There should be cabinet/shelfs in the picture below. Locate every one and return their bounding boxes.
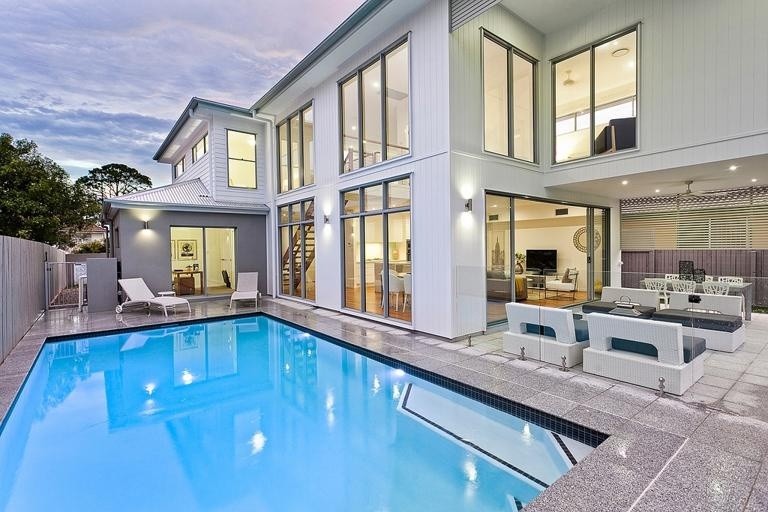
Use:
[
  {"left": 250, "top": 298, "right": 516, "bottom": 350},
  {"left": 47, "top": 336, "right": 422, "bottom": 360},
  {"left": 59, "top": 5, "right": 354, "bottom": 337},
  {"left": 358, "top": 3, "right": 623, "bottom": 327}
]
[{"left": 175, "top": 276, "right": 195, "bottom": 295}]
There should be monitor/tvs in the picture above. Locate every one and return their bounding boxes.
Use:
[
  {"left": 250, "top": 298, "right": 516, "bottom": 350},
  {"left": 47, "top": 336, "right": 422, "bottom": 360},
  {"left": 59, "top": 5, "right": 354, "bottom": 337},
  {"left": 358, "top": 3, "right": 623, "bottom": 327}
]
[{"left": 527, "top": 249, "right": 557, "bottom": 272}]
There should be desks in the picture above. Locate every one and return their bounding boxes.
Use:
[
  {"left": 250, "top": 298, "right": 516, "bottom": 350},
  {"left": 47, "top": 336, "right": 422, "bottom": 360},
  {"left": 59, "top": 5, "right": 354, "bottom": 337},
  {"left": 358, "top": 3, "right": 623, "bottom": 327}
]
[{"left": 174, "top": 271, "right": 204, "bottom": 294}]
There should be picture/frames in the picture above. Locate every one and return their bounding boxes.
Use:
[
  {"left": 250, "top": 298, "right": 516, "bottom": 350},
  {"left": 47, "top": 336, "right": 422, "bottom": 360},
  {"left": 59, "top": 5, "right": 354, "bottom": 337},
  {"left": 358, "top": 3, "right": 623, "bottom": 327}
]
[
  {"left": 171, "top": 240, "right": 177, "bottom": 261},
  {"left": 178, "top": 240, "right": 197, "bottom": 260}
]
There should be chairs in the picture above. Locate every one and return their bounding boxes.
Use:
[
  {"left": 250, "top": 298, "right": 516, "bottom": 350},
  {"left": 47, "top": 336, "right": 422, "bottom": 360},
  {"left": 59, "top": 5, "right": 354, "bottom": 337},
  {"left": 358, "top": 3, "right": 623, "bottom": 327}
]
[
  {"left": 379, "top": 268, "right": 412, "bottom": 313},
  {"left": 502, "top": 261, "right": 751, "bottom": 398},
  {"left": 116, "top": 279, "right": 192, "bottom": 320},
  {"left": 545, "top": 268, "right": 580, "bottom": 300},
  {"left": 593, "top": 116, "right": 635, "bottom": 156},
  {"left": 230, "top": 272, "right": 262, "bottom": 310}
]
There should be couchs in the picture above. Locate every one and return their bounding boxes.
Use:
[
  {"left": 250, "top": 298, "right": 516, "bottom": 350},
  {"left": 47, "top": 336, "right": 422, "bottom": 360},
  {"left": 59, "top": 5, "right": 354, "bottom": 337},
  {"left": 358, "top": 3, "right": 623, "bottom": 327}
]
[{"left": 486, "top": 271, "right": 528, "bottom": 302}]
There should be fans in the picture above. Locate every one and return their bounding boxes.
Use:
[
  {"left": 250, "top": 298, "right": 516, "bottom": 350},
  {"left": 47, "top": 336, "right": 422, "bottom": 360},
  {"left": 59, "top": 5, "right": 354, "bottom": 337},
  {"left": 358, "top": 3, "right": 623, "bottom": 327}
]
[
  {"left": 562, "top": 70, "right": 574, "bottom": 87},
  {"left": 662, "top": 179, "right": 729, "bottom": 200}
]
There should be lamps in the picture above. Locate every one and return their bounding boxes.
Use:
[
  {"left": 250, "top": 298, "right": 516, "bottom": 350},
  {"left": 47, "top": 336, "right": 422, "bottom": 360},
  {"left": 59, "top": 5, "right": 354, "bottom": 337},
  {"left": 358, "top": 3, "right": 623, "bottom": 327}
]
[
  {"left": 465, "top": 198, "right": 473, "bottom": 212},
  {"left": 144, "top": 222, "right": 148, "bottom": 229}
]
